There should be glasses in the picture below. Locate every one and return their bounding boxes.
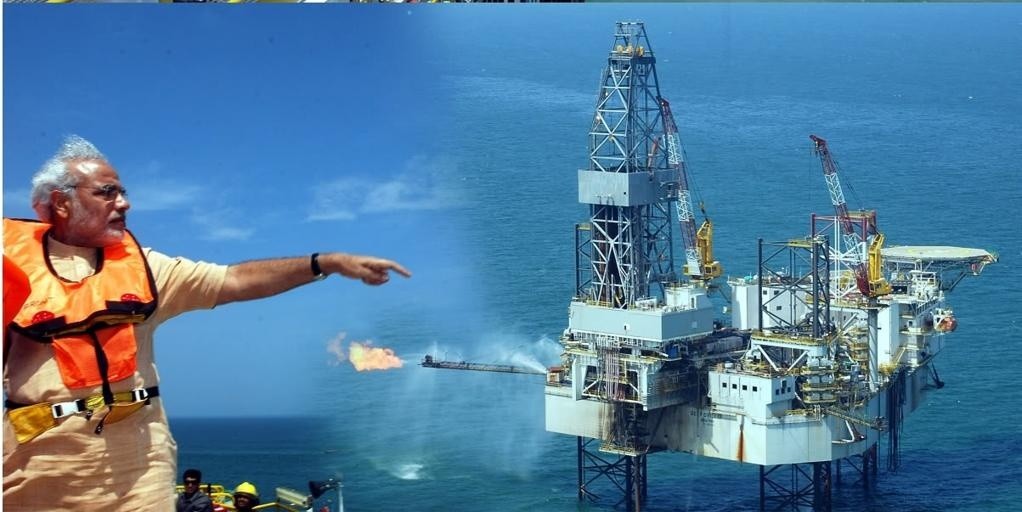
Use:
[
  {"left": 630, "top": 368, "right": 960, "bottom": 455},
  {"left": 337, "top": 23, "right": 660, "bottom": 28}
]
[{"left": 62, "top": 183, "right": 128, "bottom": 202}]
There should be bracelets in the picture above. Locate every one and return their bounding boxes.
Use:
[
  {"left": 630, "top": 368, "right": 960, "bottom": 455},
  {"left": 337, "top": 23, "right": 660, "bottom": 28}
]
[{"left": 310, "top": 252, "right": 328, "bottom": 280}]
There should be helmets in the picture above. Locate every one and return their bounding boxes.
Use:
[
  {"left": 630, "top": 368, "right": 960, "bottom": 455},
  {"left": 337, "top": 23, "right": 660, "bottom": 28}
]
[{"left": 234, "top": 482, "right": 257, "bottom": 497}]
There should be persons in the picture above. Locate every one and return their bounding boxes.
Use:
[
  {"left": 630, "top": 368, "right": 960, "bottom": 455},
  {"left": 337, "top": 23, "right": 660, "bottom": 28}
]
[
  {"left": 230, "top": 481, "right": 258, "bottom": 511},
  {"left": 4, "top": 134, "right": 411, "bottom": 512},
  {"left": 175, "top": 469, "right": 213, "bottom": 512}
]
